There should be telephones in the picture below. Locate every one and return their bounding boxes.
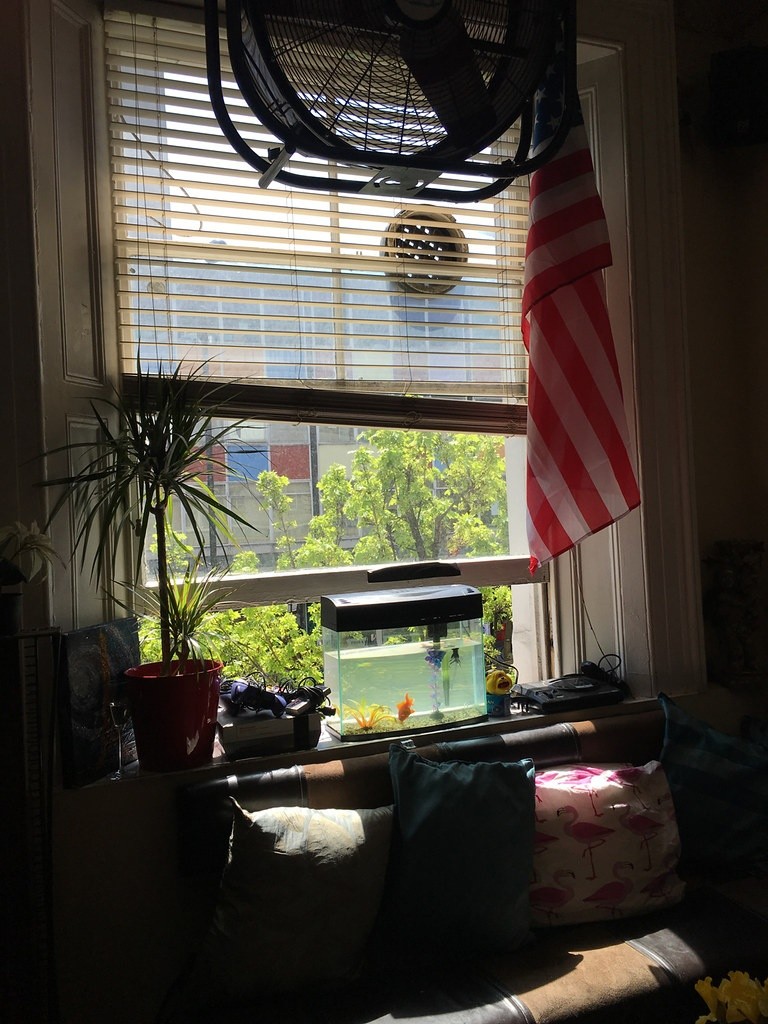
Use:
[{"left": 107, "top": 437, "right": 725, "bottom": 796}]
[{"left": 512, "top": 661, "right": 631, "bottom": 712}]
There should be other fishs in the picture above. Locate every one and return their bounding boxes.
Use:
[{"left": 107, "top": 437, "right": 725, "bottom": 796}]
[
  {"left": 397, "top": 692, "right": 416, "bottom": 721},
  {"left": 449, "top": 648, "right": 461, "bottom": 666}
]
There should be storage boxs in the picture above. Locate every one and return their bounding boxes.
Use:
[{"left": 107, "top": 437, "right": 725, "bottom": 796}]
[{"left": 320, "top": 583, "right": 488, "bottom": 742}]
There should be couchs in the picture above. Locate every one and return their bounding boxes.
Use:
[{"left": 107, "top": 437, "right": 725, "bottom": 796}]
[{"left": 172, "top": 708, "right": 767, "bottom": 1024}]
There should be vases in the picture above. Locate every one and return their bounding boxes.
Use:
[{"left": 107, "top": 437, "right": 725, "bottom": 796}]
[{"left": 0, "top": 593, "right": 25, "bottom": 634}]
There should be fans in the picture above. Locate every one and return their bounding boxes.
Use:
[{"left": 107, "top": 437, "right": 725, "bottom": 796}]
[{"left": 204, "top": 1, "right": 576, "bottom": 202}]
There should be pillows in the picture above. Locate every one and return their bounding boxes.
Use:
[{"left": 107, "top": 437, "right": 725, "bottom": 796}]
[
  {"left": 529, "top": 761, "right": 685, "bottom": 923},
  {"left": 389, "top": 741, "right": 538, "bottom": 948},
  {"left": 209, "top": 800, "right": 395, "bottom": 984}
]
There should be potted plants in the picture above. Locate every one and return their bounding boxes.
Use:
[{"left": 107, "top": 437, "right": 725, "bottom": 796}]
[
  {"left": 28, "top": 346, "right": 271, "bottom": 772},
  {"left": 483, "top": 585, "right": 513, "bottom": 640}
]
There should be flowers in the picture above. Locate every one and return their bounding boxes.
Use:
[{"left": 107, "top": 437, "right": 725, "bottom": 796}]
[
  {"left": 693, "top": 970, "right": 768, "bottom": 1024},
  {"left": 0, "top": 521, "right": 68, "bottom": 592}
]
